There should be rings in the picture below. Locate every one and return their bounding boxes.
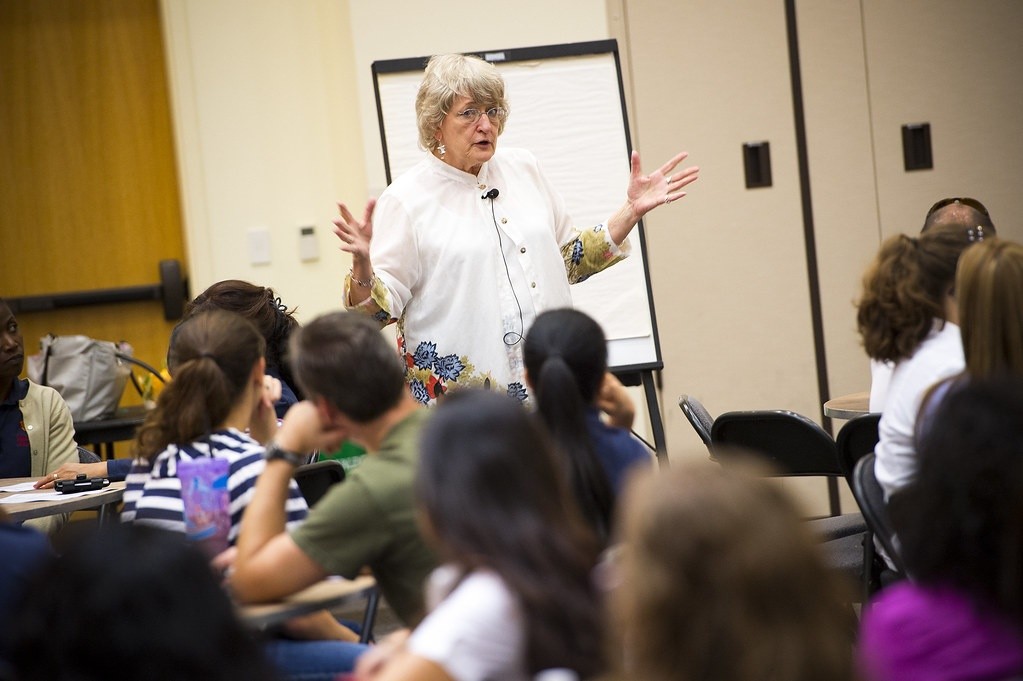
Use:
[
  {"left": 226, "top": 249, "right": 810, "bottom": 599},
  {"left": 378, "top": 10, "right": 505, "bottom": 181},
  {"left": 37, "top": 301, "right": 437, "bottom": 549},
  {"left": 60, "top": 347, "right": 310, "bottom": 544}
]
[
  {"left": 665, "top": 196, "right": 671, "bottom": 204},
  {"left": 54, "top": 474, "right": 57, "bottom": 479},
  {"left": 666, "top": 176, "right": 672, "bottom": 184}
]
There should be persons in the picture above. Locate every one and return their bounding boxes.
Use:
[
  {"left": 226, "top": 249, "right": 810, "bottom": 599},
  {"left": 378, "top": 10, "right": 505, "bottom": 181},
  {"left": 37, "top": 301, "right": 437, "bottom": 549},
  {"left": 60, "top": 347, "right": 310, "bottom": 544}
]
[
  {"left": 0, "top": 280, "right": 856, "bottom": 681},
  {"left": 333, "top": 54, "right": 699, "bottom": 411},
  {"left": 856, "top": 198, "right": 1023, "bottom": 681}
]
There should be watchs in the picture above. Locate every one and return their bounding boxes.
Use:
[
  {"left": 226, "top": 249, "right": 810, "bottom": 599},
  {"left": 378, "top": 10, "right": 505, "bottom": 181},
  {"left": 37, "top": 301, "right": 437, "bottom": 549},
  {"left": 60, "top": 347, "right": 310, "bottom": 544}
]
[{"left": 263, "top": 441, "right": 302, "bottom": 463}]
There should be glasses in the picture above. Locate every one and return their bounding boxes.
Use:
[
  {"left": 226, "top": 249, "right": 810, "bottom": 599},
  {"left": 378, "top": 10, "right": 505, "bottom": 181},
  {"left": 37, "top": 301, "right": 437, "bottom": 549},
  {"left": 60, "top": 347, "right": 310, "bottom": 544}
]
[{"left": 446, "top": 107, "right": 504, "bottom": 124}]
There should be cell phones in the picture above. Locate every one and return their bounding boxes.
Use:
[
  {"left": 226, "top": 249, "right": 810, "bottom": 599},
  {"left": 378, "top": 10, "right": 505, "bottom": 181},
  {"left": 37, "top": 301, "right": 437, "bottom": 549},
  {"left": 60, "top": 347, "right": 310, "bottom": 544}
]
[{"left": 55, "top": 479, "right": 110, "bottom": 493}]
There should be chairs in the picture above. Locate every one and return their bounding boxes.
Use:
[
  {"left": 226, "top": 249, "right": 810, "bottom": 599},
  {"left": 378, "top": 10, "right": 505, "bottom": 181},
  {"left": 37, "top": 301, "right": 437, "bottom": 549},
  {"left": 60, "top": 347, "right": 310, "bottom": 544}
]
[
  {"left": 294, "top": 460, "right": 390, "bottom": 651},
  {"left": 678, "top": 396, "right": 907, "bottom": 645}
]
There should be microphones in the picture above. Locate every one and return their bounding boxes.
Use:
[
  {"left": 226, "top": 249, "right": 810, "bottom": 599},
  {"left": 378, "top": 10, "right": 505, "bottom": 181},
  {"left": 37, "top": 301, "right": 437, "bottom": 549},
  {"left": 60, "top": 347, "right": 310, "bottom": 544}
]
[{"left": 487, "top": 189, "right": 499, "bottom": 199}]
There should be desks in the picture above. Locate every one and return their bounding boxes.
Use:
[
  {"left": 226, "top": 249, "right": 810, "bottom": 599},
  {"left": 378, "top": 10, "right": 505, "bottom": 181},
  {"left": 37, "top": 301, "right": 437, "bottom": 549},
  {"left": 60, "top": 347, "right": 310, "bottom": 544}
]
[
  {"left": 0, "top": 477, "right": 125, "bottom": 538},
  {"left": 822, "top": 394, "right": 870, "bottom": 420},
  {"left": 239, "top": 573, "right": 381, "bottom": 633},
  {"left": 69, "top": 404, "right": 159, "bottom": 463}
]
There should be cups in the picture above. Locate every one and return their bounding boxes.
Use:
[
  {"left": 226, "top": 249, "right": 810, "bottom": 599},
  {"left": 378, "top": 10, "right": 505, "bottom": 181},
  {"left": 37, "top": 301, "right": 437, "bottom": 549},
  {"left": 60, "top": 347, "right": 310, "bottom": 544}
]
[{"left": 176, "top": 458, "right": 231, "bottom": 557}]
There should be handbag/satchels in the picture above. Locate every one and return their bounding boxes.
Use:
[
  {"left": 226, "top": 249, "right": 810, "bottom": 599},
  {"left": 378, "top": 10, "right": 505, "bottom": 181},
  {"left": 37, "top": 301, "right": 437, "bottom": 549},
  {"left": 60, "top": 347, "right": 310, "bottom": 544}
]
[{"left": 25, "top": 332, "right": 168, "bottom": 423}]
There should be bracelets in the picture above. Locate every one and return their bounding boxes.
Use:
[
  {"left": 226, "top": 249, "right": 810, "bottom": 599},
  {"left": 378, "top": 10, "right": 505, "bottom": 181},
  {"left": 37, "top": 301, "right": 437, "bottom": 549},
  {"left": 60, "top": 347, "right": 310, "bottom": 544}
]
[{"left": 350, "top": 269, "right": 376, "bottom": 287}]
